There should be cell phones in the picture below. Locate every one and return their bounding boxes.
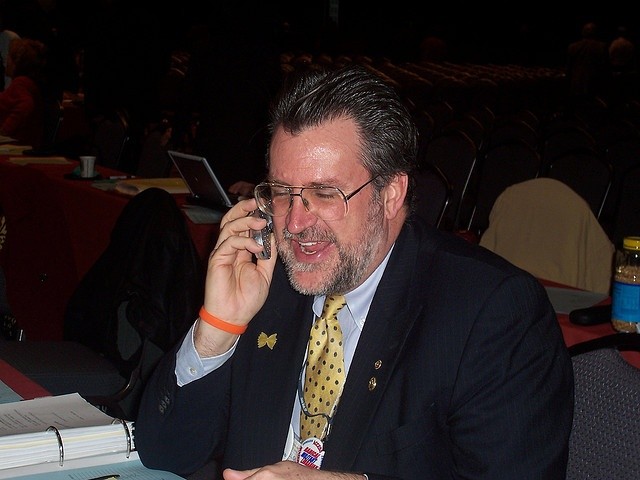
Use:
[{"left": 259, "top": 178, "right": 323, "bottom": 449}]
[{"left": 248, "top": 199, "right": 273, "bottom": 260}]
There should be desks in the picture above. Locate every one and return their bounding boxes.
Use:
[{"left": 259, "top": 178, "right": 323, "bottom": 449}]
[
  {"left": 539, "top": 276, "right": 640, "bottom": 372},
  {"left": 1, "top": 133, "right": 222, "bottom": 342},
  {"left": 0, "top": 362, "right": 53, "bottom": 400}
]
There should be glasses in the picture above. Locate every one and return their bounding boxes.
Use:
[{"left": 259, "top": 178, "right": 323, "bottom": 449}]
[{"left": 254, "top": 172, "right": 384, "bottom": 221}]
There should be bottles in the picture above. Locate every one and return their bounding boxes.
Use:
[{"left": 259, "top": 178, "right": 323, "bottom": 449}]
[{"left": 612, "top": 236, "right": 640, "bottom": 333}]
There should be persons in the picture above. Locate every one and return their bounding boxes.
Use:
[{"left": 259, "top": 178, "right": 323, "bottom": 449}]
[
  {"left": 134, "top": 65, "right": 574, "bottom": 478},
  {"left": 0, "top": 39, "right": 47, "bottom": 136},
  {"left": 23, "top": 68, "right": 126, "bottom": 170}
]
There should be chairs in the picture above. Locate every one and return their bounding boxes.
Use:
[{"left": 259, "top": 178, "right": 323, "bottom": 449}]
[
  {"left": 278, "top": 50, "right": 595, "bottom": 104},
  {"left": 2, "top": 337, "right": 147, "bottom": 397},
  {"left": 564, "top": 347, "right": 640, "bottom": 480},
  {"left": 405, "top": 99, "right": 629, "bottom": 231}
]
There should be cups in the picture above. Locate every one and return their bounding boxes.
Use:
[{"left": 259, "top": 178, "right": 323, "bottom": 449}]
[{"left": 79, "top": 155, "right": 96, "bottom": 180}]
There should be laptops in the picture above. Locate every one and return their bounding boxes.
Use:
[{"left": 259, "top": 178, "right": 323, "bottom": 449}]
[{"left": 168, "top": 150, "right": 254, "bottom": 211}]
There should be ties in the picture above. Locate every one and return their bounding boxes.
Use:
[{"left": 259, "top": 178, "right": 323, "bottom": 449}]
[{"left": 300, "top": 296, "right": 346, "bottom": 443}]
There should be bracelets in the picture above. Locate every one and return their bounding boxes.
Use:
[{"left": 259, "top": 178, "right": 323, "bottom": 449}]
[{"left": 199, "top": 307, "right": 251, "bottom": 336}]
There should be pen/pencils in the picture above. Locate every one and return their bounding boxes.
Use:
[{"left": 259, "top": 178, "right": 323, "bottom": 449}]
[
  {"left": 107, "top": 175, "right": 136, "bottom": 180},
  {"left": 86, "top": 474, "right": 120, "bottom": 480}
]
